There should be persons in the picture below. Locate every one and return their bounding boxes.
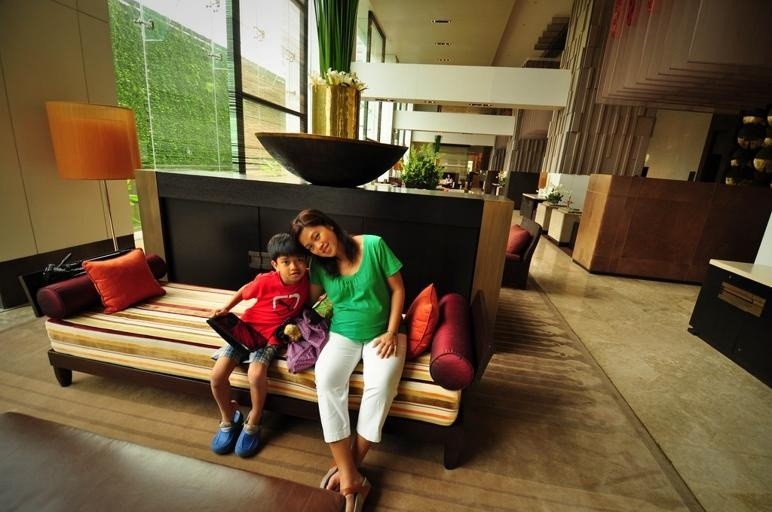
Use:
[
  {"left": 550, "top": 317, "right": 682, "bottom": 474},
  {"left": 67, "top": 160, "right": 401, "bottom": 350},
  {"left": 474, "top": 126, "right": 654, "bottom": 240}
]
[
  {"left": 207, "top": 235, "right": 315, "bottom": 458},
  {"left": 288, "top": 209, "right": 408, "bottom": 510}
]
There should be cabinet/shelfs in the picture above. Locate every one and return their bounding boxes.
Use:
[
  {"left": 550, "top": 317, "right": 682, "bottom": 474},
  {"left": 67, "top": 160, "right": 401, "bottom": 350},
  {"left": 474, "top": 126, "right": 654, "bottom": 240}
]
[
  {"left": 695, "top": 113, "right": 772, "bottom": 184},
  {"left": 687, "top": 258, "right": 772, "bottom": 388}
]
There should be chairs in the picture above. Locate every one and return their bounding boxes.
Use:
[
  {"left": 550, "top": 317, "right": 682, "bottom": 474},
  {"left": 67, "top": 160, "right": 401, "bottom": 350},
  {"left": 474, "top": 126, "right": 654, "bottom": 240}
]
[{"left": 501, "top": 217, "right": 542, "bottom": 289}]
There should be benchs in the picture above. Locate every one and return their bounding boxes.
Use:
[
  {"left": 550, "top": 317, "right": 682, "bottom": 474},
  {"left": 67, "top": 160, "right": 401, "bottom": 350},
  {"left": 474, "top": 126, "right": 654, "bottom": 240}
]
[{"left": 19, "top": 250, "right": 495, "bottom": 469}]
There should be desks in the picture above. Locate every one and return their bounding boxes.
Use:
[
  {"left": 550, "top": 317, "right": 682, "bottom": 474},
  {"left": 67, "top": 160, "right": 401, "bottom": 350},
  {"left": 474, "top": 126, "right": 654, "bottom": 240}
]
[{"left": 523, "top": 193, "right": 582, "bottom": 247}]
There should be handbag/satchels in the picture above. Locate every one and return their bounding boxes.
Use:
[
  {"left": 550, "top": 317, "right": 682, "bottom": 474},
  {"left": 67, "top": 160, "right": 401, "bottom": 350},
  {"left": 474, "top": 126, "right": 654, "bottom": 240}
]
[{"left": 287, "top": 309, "right": 329, "bottom": 373}]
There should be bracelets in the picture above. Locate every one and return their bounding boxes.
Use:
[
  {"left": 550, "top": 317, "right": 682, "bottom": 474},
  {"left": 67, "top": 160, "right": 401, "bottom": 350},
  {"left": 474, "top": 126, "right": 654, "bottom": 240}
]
[{"left": 386, "top": 331, "right": 397, "bottom": 336}]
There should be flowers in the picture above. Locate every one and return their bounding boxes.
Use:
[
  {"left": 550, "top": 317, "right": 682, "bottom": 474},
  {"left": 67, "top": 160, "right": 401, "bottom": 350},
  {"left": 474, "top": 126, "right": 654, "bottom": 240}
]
[
  {"left": 545, "top": 185, "right": 570, "bottom": 205},
  {"left": 311, "top": 67, "right": 368, "bottom": 91}
]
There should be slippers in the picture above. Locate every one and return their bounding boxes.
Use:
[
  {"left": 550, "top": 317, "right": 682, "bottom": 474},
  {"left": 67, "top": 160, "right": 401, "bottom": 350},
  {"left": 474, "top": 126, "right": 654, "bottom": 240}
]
[
  {"left": 320, "top": 465, "right": 338, "bottom": 490},
  {"left": 341, "top": 477, "right": 372, "bottom": 512},
  {"left": 235, "top": 410, "right": 265, "bottom": 457},
  {"left": 210, "top": 410, "right": 243, "bottom": 455}
]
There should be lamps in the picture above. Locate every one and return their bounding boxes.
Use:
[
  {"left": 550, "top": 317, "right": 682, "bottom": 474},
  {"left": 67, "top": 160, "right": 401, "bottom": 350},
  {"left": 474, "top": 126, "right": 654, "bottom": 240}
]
[{"left": 47, "top": 100, "right": 143, "bottom": 252}]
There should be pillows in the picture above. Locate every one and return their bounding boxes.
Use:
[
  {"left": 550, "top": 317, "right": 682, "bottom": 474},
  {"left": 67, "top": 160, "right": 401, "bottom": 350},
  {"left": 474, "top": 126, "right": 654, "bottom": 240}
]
[
  {"left": 507, "top": 225, "right": 530, "bottom": 254},
  {"left": 82, "top": 248, "right": 166, "bottom": 315},
  {"left": 37, "top": 254, "right": 166, "bottom": 320},
  {"left": 429, "top": 293, "right": 475, "bottom": 391},
  {"left": 404, "top": 283, "right": 441, "bottom": 361}
]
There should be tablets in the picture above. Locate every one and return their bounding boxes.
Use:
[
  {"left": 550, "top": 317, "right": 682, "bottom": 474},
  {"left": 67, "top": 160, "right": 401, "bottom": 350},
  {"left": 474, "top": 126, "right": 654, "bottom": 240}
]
[{"left": 206, "top": 312, "right": 269, "bottom": 352}]
